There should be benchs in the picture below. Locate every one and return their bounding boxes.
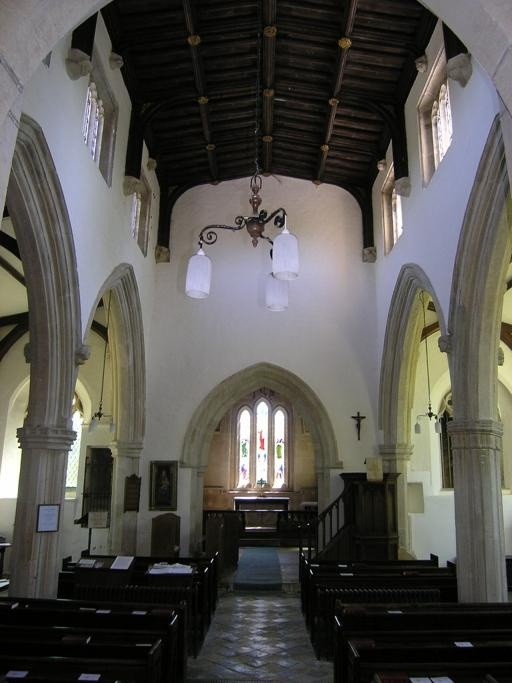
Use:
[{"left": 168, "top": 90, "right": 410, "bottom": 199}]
[
  {"left": 1, "top": 550, "right": 218, "bottom": 683},
  {"left": 298, "top": 552, "right": 512, "bottom": 682}
]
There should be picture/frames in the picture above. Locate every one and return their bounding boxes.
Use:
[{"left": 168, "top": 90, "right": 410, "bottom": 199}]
[{"left": 148, "top": 460, "right": 179, "bottom": 511}]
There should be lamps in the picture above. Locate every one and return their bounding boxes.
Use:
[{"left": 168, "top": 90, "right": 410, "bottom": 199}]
[
  {"left": 182, "top": 122, "right": 300, "bottom": 313},
  {"left": 87, "top": 290, "right": 118, "bottom": 434},
  {"left": 414, "top": 291, "right": 442, "bottom": 433}
]
